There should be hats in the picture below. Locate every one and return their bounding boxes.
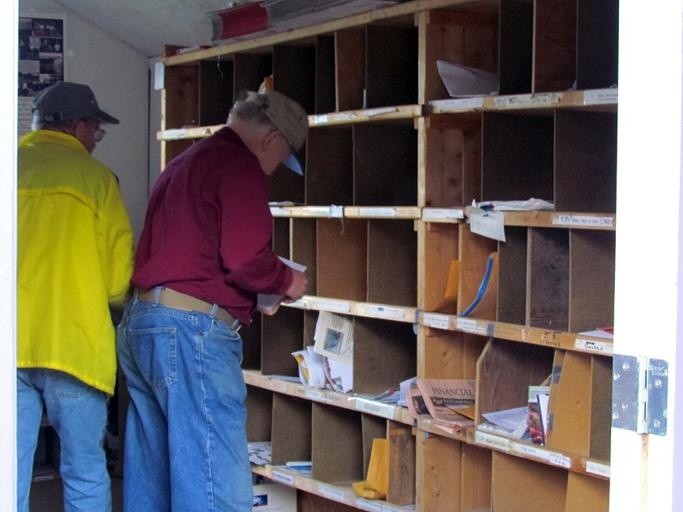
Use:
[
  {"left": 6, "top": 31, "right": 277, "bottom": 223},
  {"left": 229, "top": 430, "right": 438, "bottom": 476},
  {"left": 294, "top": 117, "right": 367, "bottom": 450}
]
[
  {"left": 249, "top": 91, "right": 309, "bottom": 176},
  {"left": 30, "top": 81, "right": 119, "bottom": 124}
]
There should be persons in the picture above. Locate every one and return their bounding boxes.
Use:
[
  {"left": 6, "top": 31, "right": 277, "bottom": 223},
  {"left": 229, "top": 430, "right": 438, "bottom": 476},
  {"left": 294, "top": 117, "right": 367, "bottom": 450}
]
[
  {"left": 39, "top": 37, "right": 51, "bottom": 54},
  {"left": 17, "top": 80, "right": 136, "bottom": 510},
  {"left": 111, "top": 88, "right": 312, "bottom": 511},
  {"left": 27, "top": 29, "right": 40, "bottom": 61}
]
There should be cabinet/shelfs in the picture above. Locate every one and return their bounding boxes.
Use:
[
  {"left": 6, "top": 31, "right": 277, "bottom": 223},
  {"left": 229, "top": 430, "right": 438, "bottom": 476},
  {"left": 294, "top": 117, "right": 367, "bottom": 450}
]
[{"left": 155, "top": 0, "right": 620, "bottom": 512}]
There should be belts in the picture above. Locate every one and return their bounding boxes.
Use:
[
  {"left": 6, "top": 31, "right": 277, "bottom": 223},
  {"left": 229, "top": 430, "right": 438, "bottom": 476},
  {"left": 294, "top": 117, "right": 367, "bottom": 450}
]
[{"left": 138, "top": 286, "right": 243, "bottom": 333}]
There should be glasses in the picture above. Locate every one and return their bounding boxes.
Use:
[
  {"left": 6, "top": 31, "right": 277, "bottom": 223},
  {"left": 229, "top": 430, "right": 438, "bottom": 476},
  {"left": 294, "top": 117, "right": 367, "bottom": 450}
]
[{"left": 94, "top": 126, "right": 105, "bottom": 142}]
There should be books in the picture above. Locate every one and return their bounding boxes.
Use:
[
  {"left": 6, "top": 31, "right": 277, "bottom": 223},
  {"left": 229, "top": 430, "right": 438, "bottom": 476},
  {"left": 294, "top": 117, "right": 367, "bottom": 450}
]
[{"left": 253, "top": 254, "right": 311, "bottom": 317}]
